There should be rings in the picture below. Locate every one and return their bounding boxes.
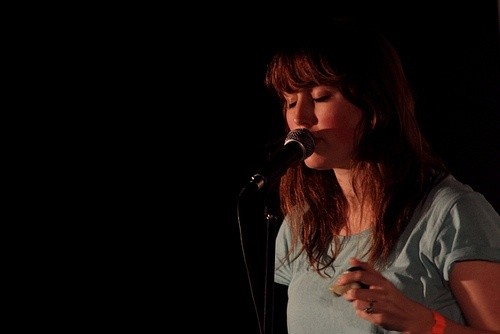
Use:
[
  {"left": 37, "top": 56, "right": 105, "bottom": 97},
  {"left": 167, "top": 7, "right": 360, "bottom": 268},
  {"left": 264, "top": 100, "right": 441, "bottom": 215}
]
[{"left": 364, "top": 303, "right": 377, "bottom": 313}]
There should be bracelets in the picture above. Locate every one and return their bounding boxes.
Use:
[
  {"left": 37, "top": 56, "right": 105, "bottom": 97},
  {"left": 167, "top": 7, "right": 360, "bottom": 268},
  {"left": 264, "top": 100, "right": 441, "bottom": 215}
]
[{"left": 432, "top": 309, "right": 446, "bottom": 334}]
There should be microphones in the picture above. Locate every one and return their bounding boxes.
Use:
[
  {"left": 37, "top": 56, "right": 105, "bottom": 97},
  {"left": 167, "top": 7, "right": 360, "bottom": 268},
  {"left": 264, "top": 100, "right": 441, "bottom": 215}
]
[{"left": 249, "top": 128, "right": 316, "bottom": 190}]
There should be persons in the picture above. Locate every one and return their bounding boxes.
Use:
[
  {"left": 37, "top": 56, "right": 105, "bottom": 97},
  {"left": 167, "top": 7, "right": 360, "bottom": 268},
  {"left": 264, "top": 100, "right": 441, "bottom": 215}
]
[{"left": 264, "top": 20, "right": 500, "bottom": 334}]
